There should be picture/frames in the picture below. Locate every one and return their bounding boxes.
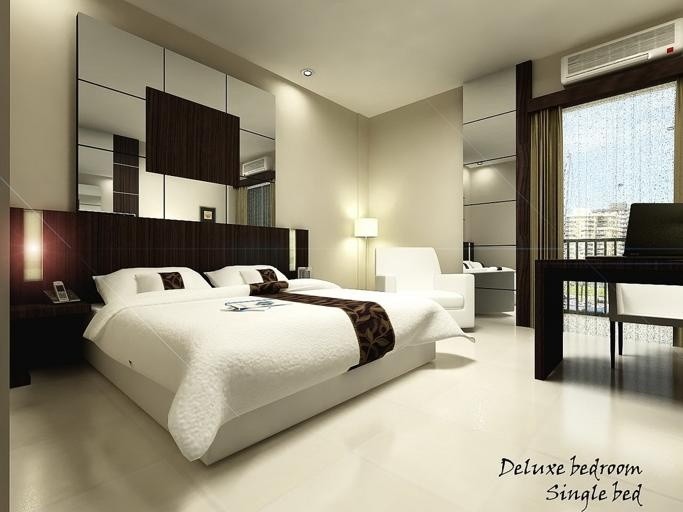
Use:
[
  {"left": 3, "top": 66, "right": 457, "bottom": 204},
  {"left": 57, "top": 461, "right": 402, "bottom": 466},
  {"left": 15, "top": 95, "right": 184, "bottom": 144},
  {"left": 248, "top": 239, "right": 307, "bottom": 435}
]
[{"left": 199, "top": 205, "right": 215, "bottom": 223}]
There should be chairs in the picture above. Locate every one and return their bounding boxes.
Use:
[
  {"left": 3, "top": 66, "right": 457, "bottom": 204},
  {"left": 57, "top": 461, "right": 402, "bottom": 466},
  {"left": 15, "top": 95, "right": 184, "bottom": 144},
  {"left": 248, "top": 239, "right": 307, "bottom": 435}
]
[
  {"left": 463, "top": 256, "right": 516, "bottom": 316},
  {"left": 372, "top": 243, "right": 475, "bottom": 330},
  {"left": 608, "top": 202, "right": 683, "bottom": 370}
]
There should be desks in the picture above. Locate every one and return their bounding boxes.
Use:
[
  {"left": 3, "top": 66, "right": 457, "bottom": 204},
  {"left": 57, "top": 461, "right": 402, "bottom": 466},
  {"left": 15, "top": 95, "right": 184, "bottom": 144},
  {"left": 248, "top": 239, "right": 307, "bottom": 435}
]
[
  {"left": 534, "top": 258, "right": 682, "bottom": 381},
  {"left": 10, "top": 300, "right": 91, "bottom": 390}
]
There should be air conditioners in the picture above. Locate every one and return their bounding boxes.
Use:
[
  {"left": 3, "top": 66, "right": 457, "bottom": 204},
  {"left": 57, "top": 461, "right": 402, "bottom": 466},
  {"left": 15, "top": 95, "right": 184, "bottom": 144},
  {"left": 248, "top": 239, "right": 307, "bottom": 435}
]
[
  {"left": 241, "top": 156, "right": 272, "bottom": 176},
  {"left": 559, "top": 16, "right": 683, "bottom": 89}
]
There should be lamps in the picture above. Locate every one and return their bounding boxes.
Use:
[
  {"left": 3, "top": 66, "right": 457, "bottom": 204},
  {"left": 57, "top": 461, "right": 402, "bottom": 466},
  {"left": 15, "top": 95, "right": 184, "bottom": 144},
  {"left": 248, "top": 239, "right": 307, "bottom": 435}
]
[{"left": 353, "top": 217, "right": 379, "bottom": 290}]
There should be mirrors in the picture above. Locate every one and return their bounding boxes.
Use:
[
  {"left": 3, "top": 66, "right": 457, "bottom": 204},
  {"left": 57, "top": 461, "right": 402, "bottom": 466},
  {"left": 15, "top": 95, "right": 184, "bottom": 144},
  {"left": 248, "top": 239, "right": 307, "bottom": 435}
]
[
  {"left": 73, "top": 10, "right": 278, "bottom": 228},
  {"left": 463, "top": 63, "right": 518, "bottom": 326}
]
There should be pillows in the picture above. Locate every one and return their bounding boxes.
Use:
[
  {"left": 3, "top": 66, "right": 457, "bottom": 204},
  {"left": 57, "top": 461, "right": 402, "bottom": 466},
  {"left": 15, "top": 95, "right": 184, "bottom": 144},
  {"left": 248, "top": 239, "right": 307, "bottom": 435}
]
[
  {"left": 201, "top": 263, "right": 289, "bottom": 288},
  {"left": 237, "top": 268, "right": 279, "bottom": 285},
  {"left": 132, "top": 271, "right": 185, "bottom": 295},
  {"left": 90, "top": 266, "right": 213, "bottom": 305}
]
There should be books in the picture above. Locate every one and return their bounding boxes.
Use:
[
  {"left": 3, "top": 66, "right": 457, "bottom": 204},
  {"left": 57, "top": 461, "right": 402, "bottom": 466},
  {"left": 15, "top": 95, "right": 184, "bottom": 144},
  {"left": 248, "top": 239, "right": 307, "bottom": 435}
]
[{"left": 221, "top": 298, "right": 288, "bottom": 310}]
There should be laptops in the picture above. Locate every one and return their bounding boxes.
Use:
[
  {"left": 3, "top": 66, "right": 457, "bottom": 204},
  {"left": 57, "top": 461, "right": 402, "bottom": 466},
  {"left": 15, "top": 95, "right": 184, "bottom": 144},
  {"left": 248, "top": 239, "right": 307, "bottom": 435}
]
[{"left": 584, "top": 202, "right": 683, "bottom": 261}]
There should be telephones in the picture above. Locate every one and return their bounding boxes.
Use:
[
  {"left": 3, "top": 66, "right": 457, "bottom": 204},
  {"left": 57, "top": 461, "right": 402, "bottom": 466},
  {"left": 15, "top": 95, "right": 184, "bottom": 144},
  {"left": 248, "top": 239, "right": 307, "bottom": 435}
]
[{"left": 43, "top": 281, "right": 82, "bottom": 304}]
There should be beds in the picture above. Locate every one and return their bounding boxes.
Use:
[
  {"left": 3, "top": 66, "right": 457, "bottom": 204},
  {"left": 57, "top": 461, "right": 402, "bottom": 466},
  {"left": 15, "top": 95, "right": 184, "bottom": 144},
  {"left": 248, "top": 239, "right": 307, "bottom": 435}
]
[{"left": 79, "top": 211, "right": 477, "bottom": 470}]
